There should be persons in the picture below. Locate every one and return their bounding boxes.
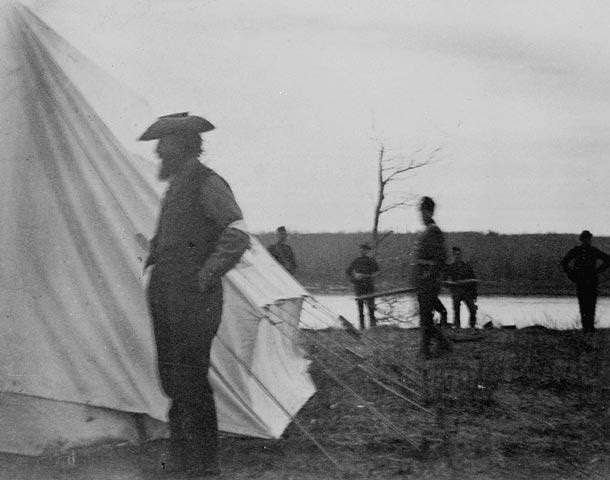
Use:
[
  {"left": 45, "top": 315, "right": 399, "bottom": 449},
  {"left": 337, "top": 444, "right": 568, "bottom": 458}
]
[
  {"left": 345, "top": 244, "right": 379, "bottom": 330},
  {"left": 139, "top": 112, "right": 252, "bottom": 480},
  {"left": 412, "top": 197, "right": 448, "bottom": 359},
  {"left": 446, "top": 247, "right": 478, "bottom": 335},
  {"left": 560, "top": 231, "right": 610, "bottom": 333},
  {"left": 267, "top": 226, "right": 296, "bottom": 275}
]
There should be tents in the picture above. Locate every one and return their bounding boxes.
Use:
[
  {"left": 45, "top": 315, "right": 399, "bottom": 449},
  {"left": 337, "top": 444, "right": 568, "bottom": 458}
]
[{"left": 0, "top": 0, "right": 317, "bottom": 457}]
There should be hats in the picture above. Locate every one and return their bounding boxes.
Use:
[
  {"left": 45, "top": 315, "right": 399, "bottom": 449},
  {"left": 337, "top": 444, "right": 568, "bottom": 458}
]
[
  {"left": 451, "top": 245, "right": 460, "bottom": 251},
  {"left": 579, "top": 230, "right": 593, "bottom": 241},
  {"left": 419, "top": 196, "right": 435, "bottom": 211},
  {"left": 358, "top": 244, "right": 371, "bottom": 250},
  {"left": 276, "top": 226, "right": 287, "bottom": 232},
  {"left": 136, "top": 111, "right": 216, "bottom": 143}
]
